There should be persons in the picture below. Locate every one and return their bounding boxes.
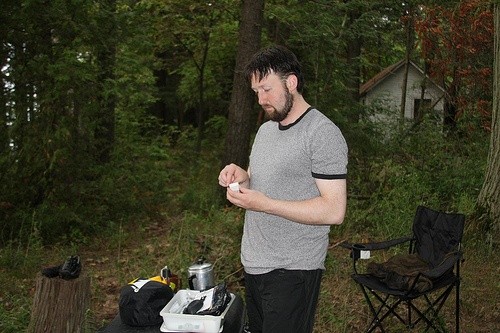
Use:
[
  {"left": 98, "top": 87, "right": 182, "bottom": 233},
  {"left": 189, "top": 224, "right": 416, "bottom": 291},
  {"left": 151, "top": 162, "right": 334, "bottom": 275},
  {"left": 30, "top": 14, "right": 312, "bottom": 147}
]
[{"left": 216, "top": 43, "right": 349, "bottom": 333}]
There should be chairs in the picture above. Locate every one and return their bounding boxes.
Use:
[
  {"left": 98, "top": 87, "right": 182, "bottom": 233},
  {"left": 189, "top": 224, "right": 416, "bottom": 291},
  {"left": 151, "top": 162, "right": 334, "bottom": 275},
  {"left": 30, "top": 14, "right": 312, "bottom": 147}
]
[{"left": 336, "top": 205, "right": 466, "bottom": 333}]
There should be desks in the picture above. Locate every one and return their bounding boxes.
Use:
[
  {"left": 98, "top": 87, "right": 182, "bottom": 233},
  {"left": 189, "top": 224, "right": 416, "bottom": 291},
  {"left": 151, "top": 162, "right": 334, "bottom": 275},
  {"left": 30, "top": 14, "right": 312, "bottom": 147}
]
[{"left": 102, "top": 290, "right": 245, "bottom": 333}]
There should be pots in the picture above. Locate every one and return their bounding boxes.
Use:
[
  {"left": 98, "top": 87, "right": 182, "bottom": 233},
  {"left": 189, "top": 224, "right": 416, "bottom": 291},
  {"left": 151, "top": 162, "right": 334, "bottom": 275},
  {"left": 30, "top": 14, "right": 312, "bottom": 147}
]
[{"left": 187, "top": 258, "right": 215, "bottom": 291}]
